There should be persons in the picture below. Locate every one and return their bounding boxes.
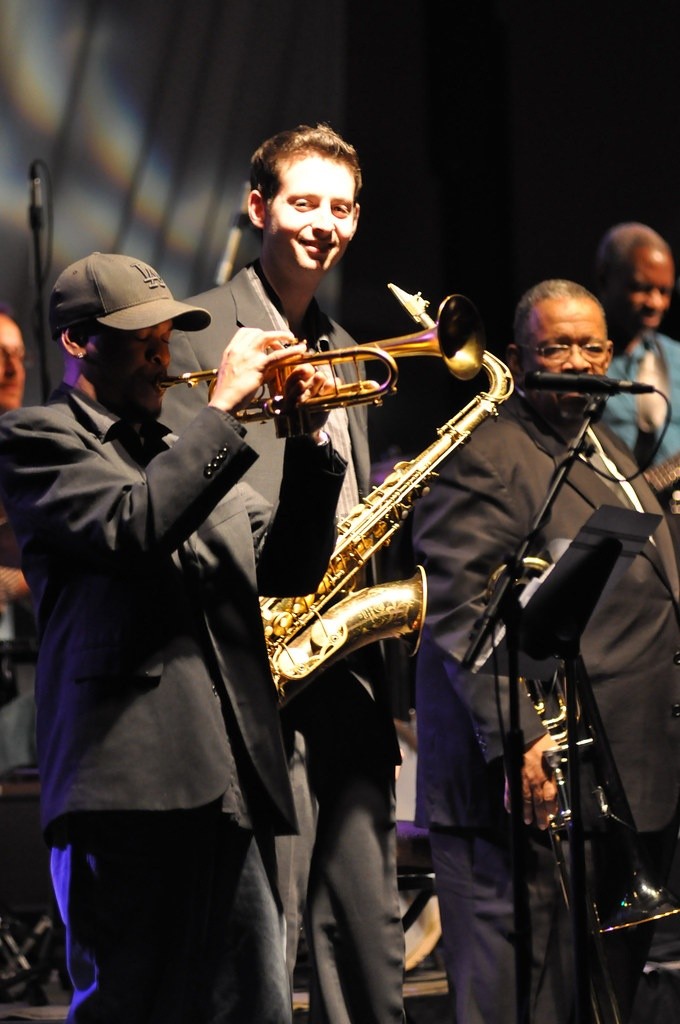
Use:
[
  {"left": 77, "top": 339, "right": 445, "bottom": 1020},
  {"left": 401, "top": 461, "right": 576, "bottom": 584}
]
[
  {"left": 158, "top": 126, "right": 423, "bottom": 1024},
  {"left": 1, "top": 222, "right": 680, "bottom": 1024}
]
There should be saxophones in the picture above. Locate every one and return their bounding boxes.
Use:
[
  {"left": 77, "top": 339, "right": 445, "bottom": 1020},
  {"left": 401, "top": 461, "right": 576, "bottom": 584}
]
[{"left": 257, "top": 282, "right": 516, "bottom": 708}]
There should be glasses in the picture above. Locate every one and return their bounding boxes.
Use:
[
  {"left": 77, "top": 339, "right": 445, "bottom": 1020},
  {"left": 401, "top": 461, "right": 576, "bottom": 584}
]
[{"left": 519, "top": 341, "right": 614, "bottom": 362}]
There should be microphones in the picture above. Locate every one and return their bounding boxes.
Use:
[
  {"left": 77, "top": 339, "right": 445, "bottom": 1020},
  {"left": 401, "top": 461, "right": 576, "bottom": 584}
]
[
  {"left": 29, "top": 164, "right": 43, "bottom": 230},
  {"left": 523, "top": 372, "right": 655, "bottom": 395}
]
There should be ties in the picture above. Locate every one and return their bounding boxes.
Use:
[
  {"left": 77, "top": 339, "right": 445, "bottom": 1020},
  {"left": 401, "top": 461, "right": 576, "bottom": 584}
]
[{"left": 581, "top": 433, "right": 637, "bottom": 511}]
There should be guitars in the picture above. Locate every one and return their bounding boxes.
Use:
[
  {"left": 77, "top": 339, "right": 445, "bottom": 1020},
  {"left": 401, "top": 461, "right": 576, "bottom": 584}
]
[{"left": 641, "top": 451, "right": 680, "bottom": 496}]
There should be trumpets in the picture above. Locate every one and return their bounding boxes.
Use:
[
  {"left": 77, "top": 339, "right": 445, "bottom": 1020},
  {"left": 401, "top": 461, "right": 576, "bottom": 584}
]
[{"left": 158, "top": 295, "right": 484, "bottom": 440}]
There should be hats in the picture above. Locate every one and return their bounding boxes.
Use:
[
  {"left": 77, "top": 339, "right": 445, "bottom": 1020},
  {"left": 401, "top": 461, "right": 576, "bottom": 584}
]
[{"left": 48, "top": 251, "right": 211, "bottom": 341}]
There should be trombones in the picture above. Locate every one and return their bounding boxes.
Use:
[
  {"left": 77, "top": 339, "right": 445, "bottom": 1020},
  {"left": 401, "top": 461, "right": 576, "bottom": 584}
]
[{"left": 485, "top": 557, "right": 680, "bottom": 1023}]
